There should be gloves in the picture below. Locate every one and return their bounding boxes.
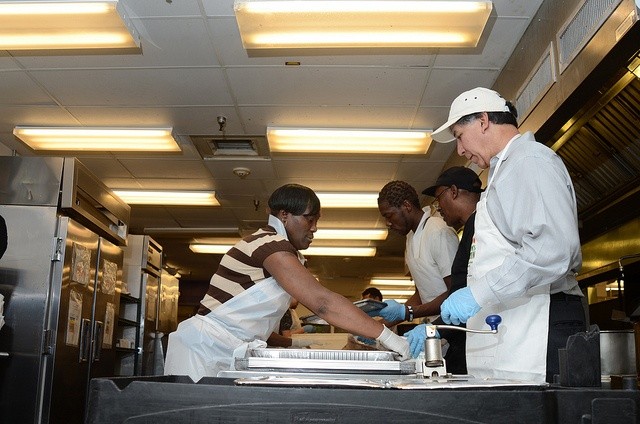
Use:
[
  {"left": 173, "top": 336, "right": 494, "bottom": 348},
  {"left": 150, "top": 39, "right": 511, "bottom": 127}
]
[
  {"left": 288, "top": 335, "right": 315, "bottom": 348},
  {"left": 376, "top": 323, "right": 409, "bottom": 362},
  {"left": 370, "top": 300, "right": 406, "bottom": 323},
  {"left": 403, "top": 324, "right": 441, "bottom": 359},
  {"left": 440, "top": 287, "right": 480, "bottom": 325},
  {"left": 303, "top": 325, "right": 315, "bottom": 331}
]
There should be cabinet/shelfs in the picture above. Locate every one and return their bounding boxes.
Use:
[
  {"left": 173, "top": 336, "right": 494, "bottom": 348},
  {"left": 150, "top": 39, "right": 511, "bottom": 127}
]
[{"left": 116, "top": 292, "right": 141, "bottom": 376}]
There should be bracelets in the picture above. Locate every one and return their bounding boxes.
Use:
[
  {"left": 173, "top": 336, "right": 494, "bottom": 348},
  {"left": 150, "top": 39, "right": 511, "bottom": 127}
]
[{"left": 407, "top": 304, "right": 414, "bottom": 323}]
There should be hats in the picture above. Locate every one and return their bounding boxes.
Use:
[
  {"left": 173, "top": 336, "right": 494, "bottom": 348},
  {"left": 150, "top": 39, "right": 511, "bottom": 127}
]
[
  {"left": 422, "top": 166, "right": 487, "bottom": 196},
  {"left": 430, "top": 87, "right": 517, "bottom": 144}
]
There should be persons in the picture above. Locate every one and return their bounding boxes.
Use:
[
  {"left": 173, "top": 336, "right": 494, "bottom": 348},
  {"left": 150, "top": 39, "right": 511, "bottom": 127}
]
[
  {"left": 362, "top": 286, "right": 383, "bottom": 301},
  {"left": 367, "top": 165, "right": 487, "bottom": 374},
  {"left": 279, "top": 295, "right": 315, "bottom": 337},
  {"left": 368, "top": 181, "right": 461, "bottom": 373},
  {"left": 429, "top": 87, "right": 590, "bottom": 384},
  {"left": 187, "top": 185, "right": 409, "bottom": 360}
]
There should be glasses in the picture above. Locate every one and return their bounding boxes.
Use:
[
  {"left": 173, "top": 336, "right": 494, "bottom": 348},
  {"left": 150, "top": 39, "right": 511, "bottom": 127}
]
[{"left": 431, "top": 187, "right": 451, "bottom": 209}]
